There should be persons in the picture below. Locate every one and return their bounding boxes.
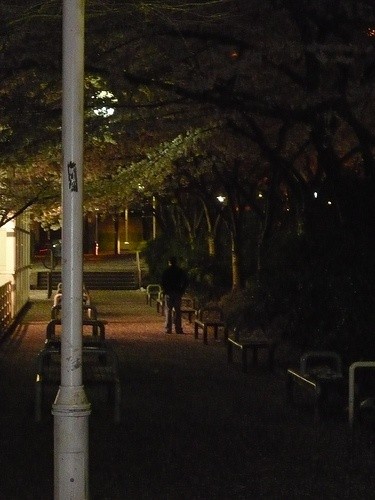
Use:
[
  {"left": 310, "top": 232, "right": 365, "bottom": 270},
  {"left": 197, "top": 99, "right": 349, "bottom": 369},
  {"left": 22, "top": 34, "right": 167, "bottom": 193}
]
[{"left": 160, "top": 256, "right": 188, "bottom": 334}]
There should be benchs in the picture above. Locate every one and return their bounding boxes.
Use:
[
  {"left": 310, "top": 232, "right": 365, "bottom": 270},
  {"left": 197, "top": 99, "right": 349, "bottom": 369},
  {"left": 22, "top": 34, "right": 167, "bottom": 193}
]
[
  {"left": 143, "top": 284, "right": 374, "bottom": 428},
  {"left": 38, "top": 278, "right": 120, "bottom": 437}
]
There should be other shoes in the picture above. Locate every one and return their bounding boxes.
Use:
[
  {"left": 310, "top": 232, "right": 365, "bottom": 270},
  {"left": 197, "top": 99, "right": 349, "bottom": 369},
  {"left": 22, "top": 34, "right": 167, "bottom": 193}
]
[
  {"left": 166, "top": 327, "right": 172, "bottom": 333},
  {"left": 176, "top": 327, "right": 184, "bottom": 334}
]
